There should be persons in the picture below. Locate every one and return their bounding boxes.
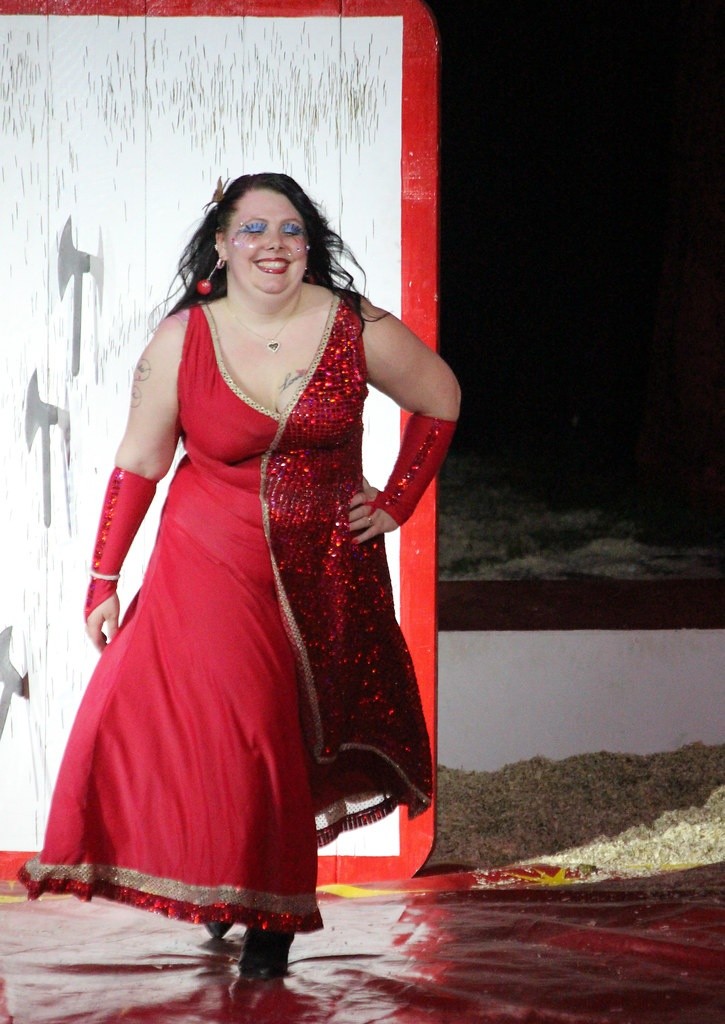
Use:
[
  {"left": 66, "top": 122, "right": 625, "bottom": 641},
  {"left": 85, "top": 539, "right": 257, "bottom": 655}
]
[{"left": 18, "top": 174, "right": 462, "bottom": 982}]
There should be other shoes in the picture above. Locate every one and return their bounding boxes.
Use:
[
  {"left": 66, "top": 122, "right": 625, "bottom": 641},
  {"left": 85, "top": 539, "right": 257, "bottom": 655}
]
[
  {"left": 237, "top": 924, "right": 293, "bottom": 978},
  {"left": 207, "top": 919, "right": 235, "bottom": 942}
]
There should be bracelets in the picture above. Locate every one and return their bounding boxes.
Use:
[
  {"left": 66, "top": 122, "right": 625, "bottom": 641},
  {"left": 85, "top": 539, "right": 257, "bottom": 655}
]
[{"left": 88, "top": 570, "right": 120, "bottom": 581}]
